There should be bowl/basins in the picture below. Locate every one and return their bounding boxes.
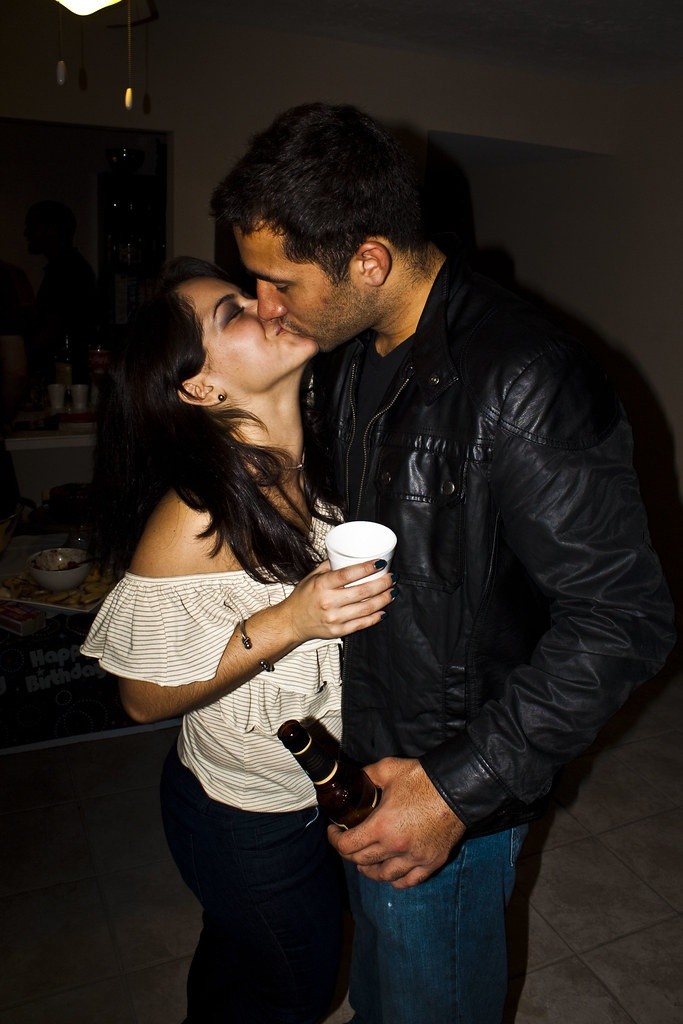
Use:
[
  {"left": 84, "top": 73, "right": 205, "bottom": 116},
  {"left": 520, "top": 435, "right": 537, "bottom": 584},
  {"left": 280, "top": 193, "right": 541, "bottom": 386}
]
[{"left": 26, "top": 548, "right": 93, "bottom": 593}]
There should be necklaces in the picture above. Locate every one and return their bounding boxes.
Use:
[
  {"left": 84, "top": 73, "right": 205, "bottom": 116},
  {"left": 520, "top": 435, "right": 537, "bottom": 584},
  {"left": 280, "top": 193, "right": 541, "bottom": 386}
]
[{"left": 249, "top": 451, "right": 306, "bottom": 471}]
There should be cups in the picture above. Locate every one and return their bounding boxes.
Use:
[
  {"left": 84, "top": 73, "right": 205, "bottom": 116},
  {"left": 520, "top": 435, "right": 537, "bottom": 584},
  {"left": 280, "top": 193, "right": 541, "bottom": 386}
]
[
  {"left": 87, "top": 344, "right": 111, "bottom": 375},
  {"left": 72, "top": 384, "right": 88, "bottom": 409},
  {"left": 48, "top": 384, "right": 65, "bottom": 409},
  {"left": 325, "top": 521, "right": 398, "bottom": 589}
]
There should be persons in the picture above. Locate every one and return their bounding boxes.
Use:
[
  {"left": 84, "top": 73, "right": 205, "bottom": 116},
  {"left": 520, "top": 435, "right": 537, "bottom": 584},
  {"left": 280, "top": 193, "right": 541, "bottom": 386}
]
[
  {"left": 209, "top": 104, "right": 679, "bottom": 1024},
  {"left": 79, "top": 255, "right": 399, "bottom": 1024},
  {"left": 0, "top": 200, "right": 97, "bottom": 431}
]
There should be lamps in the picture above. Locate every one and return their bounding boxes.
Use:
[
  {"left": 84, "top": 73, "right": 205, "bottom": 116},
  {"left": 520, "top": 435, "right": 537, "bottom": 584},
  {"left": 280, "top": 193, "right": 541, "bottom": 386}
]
[{"left": 53, "top": 0, "right": 132, "bottom": 111}]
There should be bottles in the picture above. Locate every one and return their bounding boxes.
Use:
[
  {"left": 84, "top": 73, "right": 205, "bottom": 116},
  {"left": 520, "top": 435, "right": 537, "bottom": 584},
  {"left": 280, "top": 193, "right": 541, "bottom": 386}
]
[
  {"left": 277, "top": 719, "right": 384, "bottom": 834},
  {"left": 57, "top": 334, "right": 77, "bottom": 400}
]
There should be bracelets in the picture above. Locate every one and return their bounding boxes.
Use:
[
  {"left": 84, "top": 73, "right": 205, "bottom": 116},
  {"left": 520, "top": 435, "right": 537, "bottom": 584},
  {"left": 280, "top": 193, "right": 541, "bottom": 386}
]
[{"left": 240, "top": 620, "right": 274, "bottom": 672}]
